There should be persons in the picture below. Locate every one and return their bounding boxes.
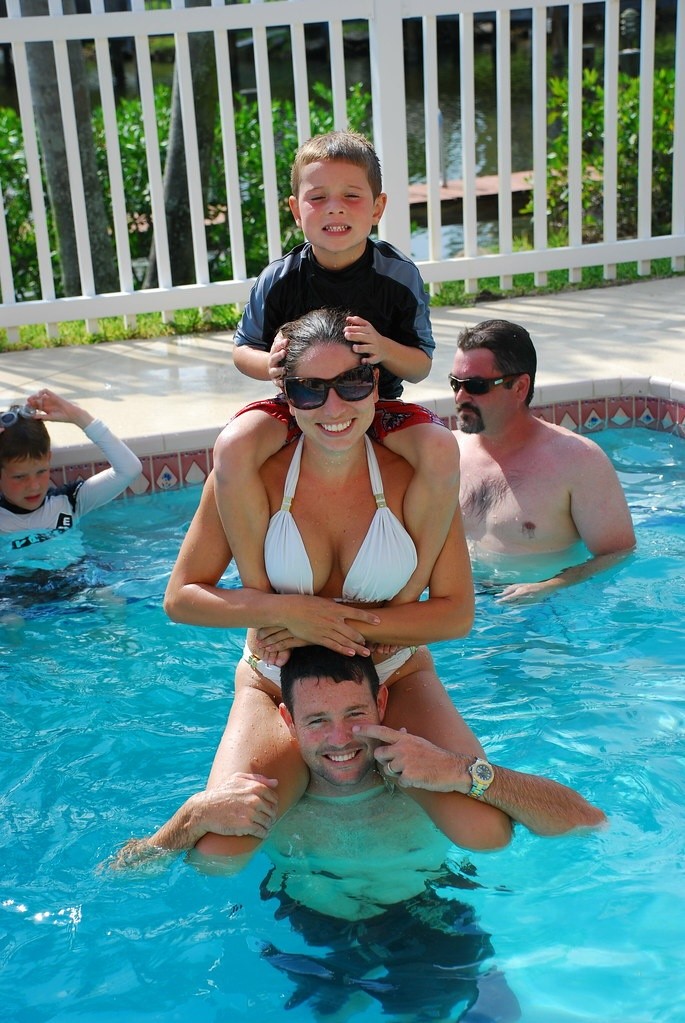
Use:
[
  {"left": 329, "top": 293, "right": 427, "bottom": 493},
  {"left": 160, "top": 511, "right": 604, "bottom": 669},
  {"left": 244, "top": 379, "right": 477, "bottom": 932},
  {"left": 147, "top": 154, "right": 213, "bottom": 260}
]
[
  {"left": 449, "top": 319, "right": 637, "bottom": 607},
  {"left": 210, "top": 127, "right": 460, "bottom": 668},
  {"left": 0, "top": 387, "right": 143, "bottom": 645},
  {"left": 163, "top": 308, "right": 515, "bottom": 876},
  {"left": 92, "top": 646, "right": 607, "bottom": 1023}
]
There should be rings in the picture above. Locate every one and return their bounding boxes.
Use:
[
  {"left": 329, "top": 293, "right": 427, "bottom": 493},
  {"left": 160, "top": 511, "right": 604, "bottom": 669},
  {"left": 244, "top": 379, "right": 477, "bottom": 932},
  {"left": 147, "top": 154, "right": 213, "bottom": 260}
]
[{"left": 385, "top": 761, "right": 399, "bottom": 777}]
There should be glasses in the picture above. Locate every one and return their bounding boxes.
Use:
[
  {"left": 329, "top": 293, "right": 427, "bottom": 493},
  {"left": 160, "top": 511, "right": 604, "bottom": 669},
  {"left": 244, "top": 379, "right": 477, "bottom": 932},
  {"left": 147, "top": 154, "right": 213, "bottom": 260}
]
[
  {"left": 0, "top": 404, "right": 38, "bottom": 428},
  {"left": 284, "top": 365, "right": 376, "bottom": 410},
  {"left": 448, "top": 374, "right": 525, "bottom": 396}
]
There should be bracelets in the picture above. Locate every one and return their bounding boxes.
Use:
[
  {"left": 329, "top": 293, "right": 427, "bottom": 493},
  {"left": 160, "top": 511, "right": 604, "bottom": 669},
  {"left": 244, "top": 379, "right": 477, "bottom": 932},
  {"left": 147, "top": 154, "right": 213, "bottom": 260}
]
[{"left": 463, "top": 753, "right": 498, "bottom": 802}]
